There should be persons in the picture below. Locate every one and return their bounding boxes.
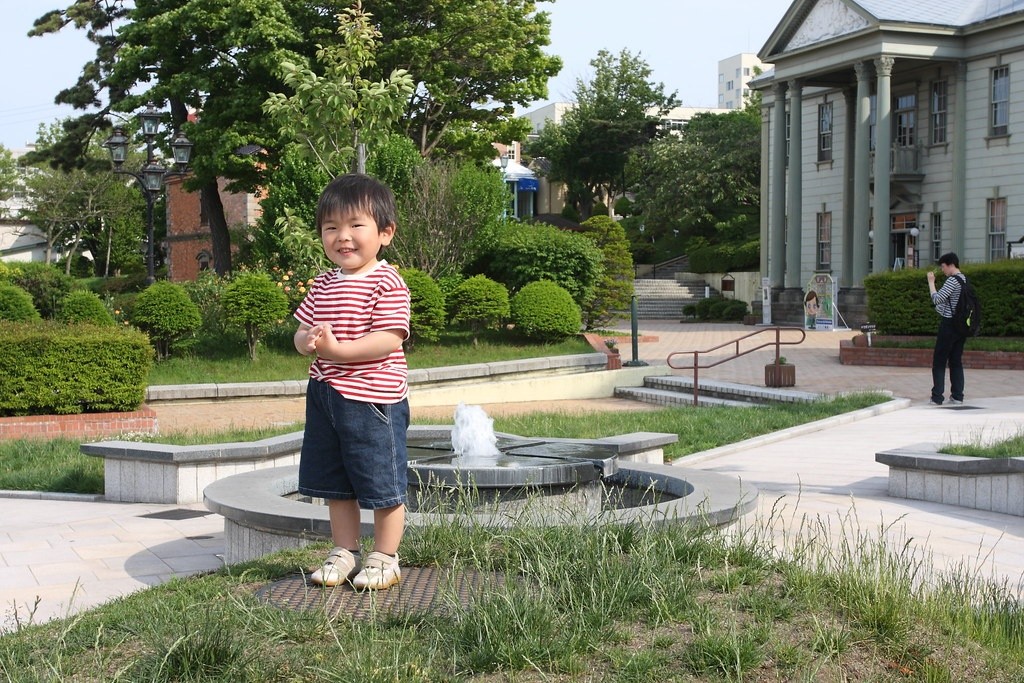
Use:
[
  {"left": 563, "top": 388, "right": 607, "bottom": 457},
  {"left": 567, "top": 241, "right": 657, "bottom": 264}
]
[
  {"left": 926, "top": 252, "right": 968, "bottom": 404},
  {"left": 291, "top": 173, "right": 412, "bottom": 587}
]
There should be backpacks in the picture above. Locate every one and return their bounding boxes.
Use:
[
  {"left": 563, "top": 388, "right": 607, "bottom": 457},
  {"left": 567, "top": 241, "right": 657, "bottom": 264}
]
[{"left": 944, "top": 274, "right": 981, "bottom": 334}]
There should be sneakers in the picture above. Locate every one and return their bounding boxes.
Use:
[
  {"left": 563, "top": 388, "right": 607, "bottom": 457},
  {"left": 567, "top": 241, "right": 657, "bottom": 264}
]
[
  {"left": 311, "top": 547, "right": 363, "bottom": 585},
  {"left": 353, "top": 552, "right": 401, "bottom": 589}
]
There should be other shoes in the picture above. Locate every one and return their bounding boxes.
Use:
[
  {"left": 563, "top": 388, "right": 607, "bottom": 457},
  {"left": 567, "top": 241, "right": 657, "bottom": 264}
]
[
  {"left": 929, "top": 397, "right": 938, "bottom": 403},
  {"left": 949, "top": 395, "right": 963, "bottom": 404}
]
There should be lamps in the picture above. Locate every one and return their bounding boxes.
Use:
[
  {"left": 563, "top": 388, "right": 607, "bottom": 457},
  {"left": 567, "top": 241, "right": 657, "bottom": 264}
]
[{"left": 910, "top": 224, "right": 925, "bottom": 236}]
